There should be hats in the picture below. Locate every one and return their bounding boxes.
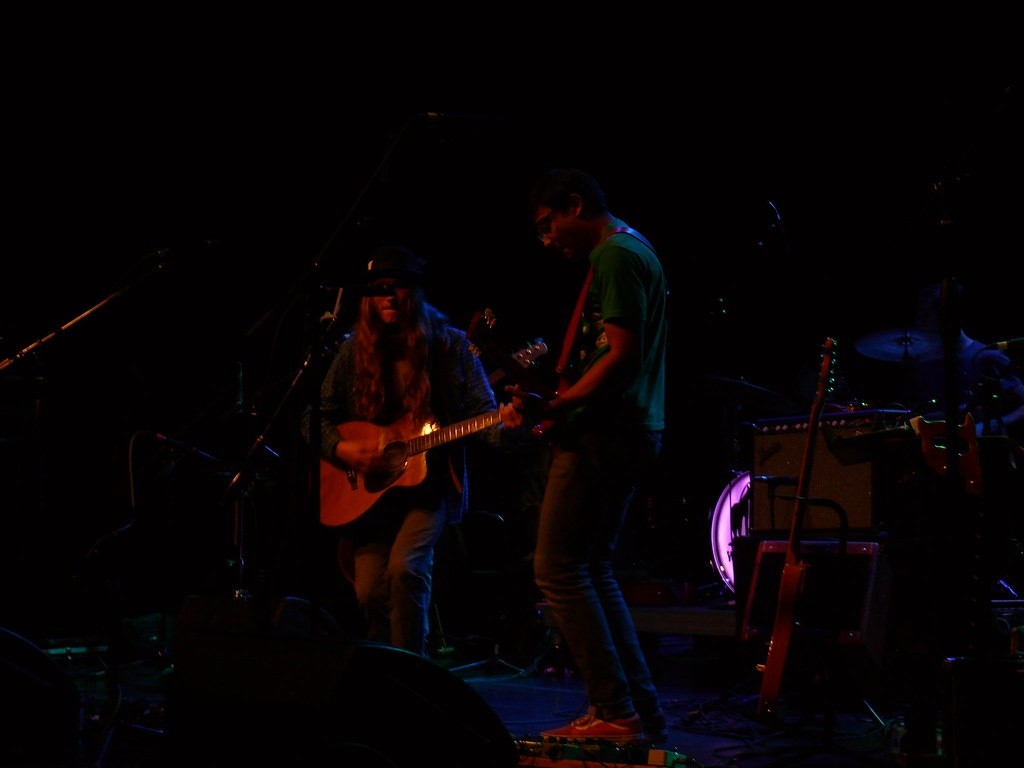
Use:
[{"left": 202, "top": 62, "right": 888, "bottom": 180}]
[{"left": 343, "top": 246, "right": 437, "bottom": 297}]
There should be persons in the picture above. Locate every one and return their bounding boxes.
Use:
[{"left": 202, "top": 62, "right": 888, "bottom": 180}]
[
  {"left": 301, "top": 244, "right": 501, "bottom": 658},
  {"left": 886, "top": 280, "right": 1024, "bottom": 436},
  {"left": 511, "top": 168, "right": 669, "bottom": 746}
]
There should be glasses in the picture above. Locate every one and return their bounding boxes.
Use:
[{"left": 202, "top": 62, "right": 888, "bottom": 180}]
[{"left": 532, "top": 207, "right": 557, "bottom": 242}]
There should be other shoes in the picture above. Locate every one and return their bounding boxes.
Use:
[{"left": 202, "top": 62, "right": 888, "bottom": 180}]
[{"left": 538, "top": 711, "right": 647, "bottom": 748}]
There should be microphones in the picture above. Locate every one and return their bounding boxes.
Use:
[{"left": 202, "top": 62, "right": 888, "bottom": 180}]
[
  {"left": 754, "top": 475, "right": 800, "bottom": 487},
  {"left": 421, "top": 110, "right": 499, "bottom": 125},
  {"left": 153, "top": 431, "right": 221, "bottom": 463}
]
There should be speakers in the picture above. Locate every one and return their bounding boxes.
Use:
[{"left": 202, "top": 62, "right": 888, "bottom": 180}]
[
  {"left": 273, "top": 596, "right": 343, "bottom": 639},
  {"left": 749, "top": 411, "right": 918, "bottom": 655},
  {"left": 326, "top": 639, "right": 513, "bottom": 768}
]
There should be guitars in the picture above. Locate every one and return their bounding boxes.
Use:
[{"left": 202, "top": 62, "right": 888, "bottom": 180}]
[
  {"left": 760, "top": 334, "right": 840, "bottom": 717},
  {"left": 320, "top": 384, "right": 553, "bottom": 527},
  {"left": 468, "top": 306, "right": 566, "bottom": 452},
  {"left": 487, "top": 332, "right": 550, "bottom": 384}
]
